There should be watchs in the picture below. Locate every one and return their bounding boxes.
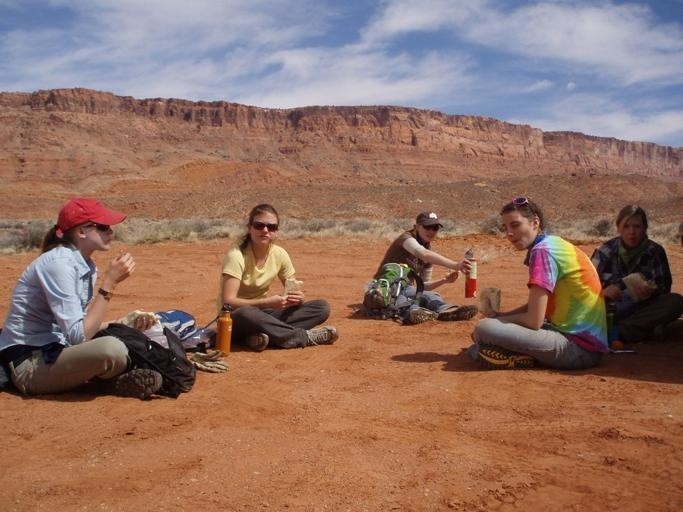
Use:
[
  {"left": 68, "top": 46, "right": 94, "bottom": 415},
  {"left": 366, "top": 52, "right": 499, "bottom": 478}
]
[{"left": 98, "top": 288, "right": 113, "bottom": 299}]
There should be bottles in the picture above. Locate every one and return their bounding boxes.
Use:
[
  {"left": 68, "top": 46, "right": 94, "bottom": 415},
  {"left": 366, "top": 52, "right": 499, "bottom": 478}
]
[
  {"left": 607, "top": 303, "right": 617, "bottom": 330},
  {"left": 465, "top": 256, "right": 478, "bottom": 299},
  {"left": 216, "top": 303, "right": 231, "bottom": 356}
]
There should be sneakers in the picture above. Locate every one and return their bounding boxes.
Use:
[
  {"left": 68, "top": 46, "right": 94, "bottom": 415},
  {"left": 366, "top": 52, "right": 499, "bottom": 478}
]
[
  {"left": 478, "top": 347, "right": 534, "bottom": 369},
  {"left": 244, "top": 333, "right": 269, "bottom": 351},
  {"left": 305, "top": 326, "right": 338, "bottom": 346},
  {"left": 411, "top": 305, "right": 478, "bottom": 324},
  {"left": 115, "top": 368, "right": 162, "bottom": 398}
]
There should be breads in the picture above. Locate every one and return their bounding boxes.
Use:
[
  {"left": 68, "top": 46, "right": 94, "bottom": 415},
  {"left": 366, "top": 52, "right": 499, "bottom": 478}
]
[
  {"left": 127, "top": 308, "right": 157, "bottom": 327},
  {"left": 284, "top": 277, "right": 303, "bottom": 297}
]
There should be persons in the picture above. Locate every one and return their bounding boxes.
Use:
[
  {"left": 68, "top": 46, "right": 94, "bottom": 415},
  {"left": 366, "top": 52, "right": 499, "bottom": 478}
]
[
  {"left": 590, "top": 204, "right": 683, "bottom": 355},
  {"left": 471, "top": 197, "right": 611, "bottom": 368},
  {"left": 372, "top": 210, "right": 478, "bottom": 325},
  {"left": 215, "top": 203, "right": 338, "bottom": 352},
  {"left": 0, "top": 197, "right": 165, "bottom": 400}
]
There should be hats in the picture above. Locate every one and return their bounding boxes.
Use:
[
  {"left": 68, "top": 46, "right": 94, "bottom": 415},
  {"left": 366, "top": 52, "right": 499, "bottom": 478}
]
[
  {"left": 56, "top": 198, "right": 127, "bottom": 236},
  {"left": 418, "top": 212, "right": 443, "bottom": 228}
]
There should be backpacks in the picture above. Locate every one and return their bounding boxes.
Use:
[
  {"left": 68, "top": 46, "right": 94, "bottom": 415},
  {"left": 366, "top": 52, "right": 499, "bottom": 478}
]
[
  {"left": 90, "top": 323, "right": 195, "bottom": 398},
  {"left": 363, "top": 263, "right": 423, "bottom": 320}
]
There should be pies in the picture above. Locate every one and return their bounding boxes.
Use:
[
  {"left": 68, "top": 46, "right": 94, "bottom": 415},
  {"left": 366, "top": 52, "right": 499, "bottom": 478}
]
[
  {"left": 623, "top": 272, "right": 657, "bottom": 302},
  {"left": 446, "top": 268, "right": 459, "bottom": 282},
  {"left": 477, "top": 286, "right": 501, "bottom": 314}
]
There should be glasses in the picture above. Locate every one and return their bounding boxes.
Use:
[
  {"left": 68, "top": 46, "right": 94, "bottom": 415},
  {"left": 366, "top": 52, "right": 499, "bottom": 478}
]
[
  {"left": 513, "top": 197, "right": 535, "bottom": 217},
  {"left": 84, "top": 223, "right": 109, "bottom": 231},
  {"left": 423, "top": 225, "right": 439, "bottom": 231},
  {"left": 251, "top": 222, "right": 278, "bottom": 231}
]
